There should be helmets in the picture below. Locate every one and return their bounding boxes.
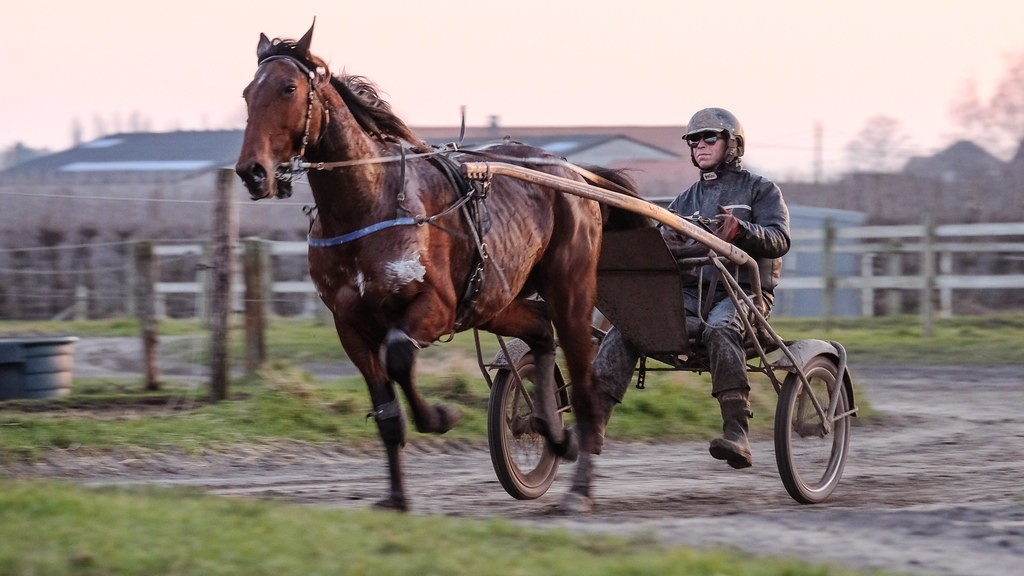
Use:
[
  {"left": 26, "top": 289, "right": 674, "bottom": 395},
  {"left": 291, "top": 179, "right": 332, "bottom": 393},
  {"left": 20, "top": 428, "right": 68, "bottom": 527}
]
[{"left": 682, "top": 107, "right": 744, "bottom": 157}]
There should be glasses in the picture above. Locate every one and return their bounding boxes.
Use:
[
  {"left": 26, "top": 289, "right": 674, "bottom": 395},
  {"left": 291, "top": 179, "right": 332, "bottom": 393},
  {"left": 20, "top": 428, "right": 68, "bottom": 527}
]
[{"left": 686, "top": 131, "right": 728, "bottom": 148}]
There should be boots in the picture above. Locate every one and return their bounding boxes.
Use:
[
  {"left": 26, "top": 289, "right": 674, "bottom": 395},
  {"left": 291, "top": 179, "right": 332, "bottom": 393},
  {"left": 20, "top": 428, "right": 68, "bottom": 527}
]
[
  {"left": 565, "top": 392, "right": 614, "bottom": 455},
  {"left": 709, "top": 397, "right": 752, "bottom": 468}
]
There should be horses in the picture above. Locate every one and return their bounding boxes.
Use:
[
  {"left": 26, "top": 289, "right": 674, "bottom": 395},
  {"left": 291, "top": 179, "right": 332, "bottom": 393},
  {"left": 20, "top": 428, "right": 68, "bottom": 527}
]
[{"left": 235, "top": 16, "right": 653, "bottom": 515}]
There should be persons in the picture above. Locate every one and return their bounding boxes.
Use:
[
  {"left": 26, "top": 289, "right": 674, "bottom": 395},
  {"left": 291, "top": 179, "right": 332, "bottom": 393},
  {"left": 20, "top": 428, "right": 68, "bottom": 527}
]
[{"left": 590, "top": 107, "right": 791, "bottom": 469}]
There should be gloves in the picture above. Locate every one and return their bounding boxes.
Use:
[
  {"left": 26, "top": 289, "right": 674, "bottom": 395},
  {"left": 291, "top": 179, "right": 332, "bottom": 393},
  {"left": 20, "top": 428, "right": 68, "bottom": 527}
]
[{"left": 712, "top": 205, "right": 739, "bottom": 242}]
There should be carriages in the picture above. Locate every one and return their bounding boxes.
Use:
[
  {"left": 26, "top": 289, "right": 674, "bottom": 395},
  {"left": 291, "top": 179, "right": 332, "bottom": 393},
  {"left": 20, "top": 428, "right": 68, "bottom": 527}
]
[{"left": 235, "top": 17, "right": 857, "bottom": 512}]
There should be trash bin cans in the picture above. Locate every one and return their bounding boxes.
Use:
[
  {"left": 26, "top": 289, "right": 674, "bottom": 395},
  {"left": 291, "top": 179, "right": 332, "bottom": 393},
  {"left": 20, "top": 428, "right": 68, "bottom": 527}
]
[{"left": 0, "top": 336, "right": 81, "bottom": 398}]
